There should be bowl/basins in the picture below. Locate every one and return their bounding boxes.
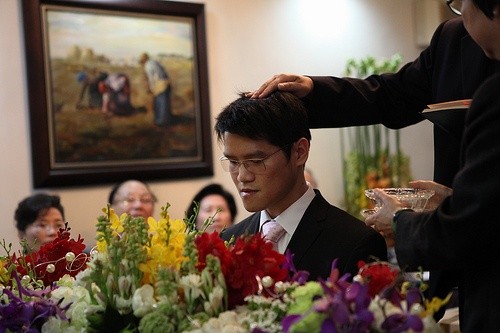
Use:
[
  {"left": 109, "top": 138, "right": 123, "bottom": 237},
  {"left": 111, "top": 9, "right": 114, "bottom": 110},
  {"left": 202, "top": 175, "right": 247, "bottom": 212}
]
[{"left": 363, "top": 187, "right": 435, "bottom": 213}]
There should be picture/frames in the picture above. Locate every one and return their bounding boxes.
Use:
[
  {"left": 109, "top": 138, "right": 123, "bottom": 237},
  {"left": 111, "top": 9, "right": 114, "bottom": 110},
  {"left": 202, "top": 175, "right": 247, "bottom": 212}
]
[{"left": 20, "top": 1, "right": 214, "bottom": 190}]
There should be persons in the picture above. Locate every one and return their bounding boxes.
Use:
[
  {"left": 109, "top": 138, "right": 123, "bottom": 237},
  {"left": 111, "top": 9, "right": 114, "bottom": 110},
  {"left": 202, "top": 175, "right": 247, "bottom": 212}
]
[
  {"left": 364, "top": 0, "right": 499, "bottom": 333},
  {"left": 214, "top": 90, "right": 388, "bottom": 281},
  {"left": 245, "top": 15, "right": 500, "bottom": 186},
  {"left": 13, "top": 193, "right": 64, "bottom": 255},
  {"left": 76, "top": 51, "right": 171, "bottom": 123},
  {"left": 108, "top": 178, "right": 157, "bottom": 222},
  {"left": 186, "top": 183, "right": 236, "bottom": 233}
]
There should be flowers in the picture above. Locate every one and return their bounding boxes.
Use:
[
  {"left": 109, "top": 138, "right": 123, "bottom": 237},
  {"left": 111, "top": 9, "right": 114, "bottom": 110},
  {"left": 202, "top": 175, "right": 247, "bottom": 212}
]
[{"left": 0, "top": 198, "right": 452, "bottom": 333}]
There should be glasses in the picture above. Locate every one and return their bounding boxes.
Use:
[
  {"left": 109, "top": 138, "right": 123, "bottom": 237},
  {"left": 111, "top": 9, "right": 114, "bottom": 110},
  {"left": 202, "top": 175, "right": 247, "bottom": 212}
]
[
  {"left": 113, "top": 197, "right": 153, "bottom": 206},
  {"left": 446, "top": 0, "right": 462, "bottom": 16},
  {"left": 218, "top": 148, "right": 279, "bottom": 175}
]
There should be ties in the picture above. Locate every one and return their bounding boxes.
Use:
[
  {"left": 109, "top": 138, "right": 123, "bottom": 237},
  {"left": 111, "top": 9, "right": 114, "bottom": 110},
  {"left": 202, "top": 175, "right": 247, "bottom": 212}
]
[{"left": 262, "top": 222, "right": 287, "bottom": 253}]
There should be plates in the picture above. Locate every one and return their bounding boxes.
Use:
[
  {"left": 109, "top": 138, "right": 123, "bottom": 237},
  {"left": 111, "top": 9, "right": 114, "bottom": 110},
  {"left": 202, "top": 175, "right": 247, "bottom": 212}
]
[{"left": 360, "top": 209, "right": 378, "bottom": 218}]
